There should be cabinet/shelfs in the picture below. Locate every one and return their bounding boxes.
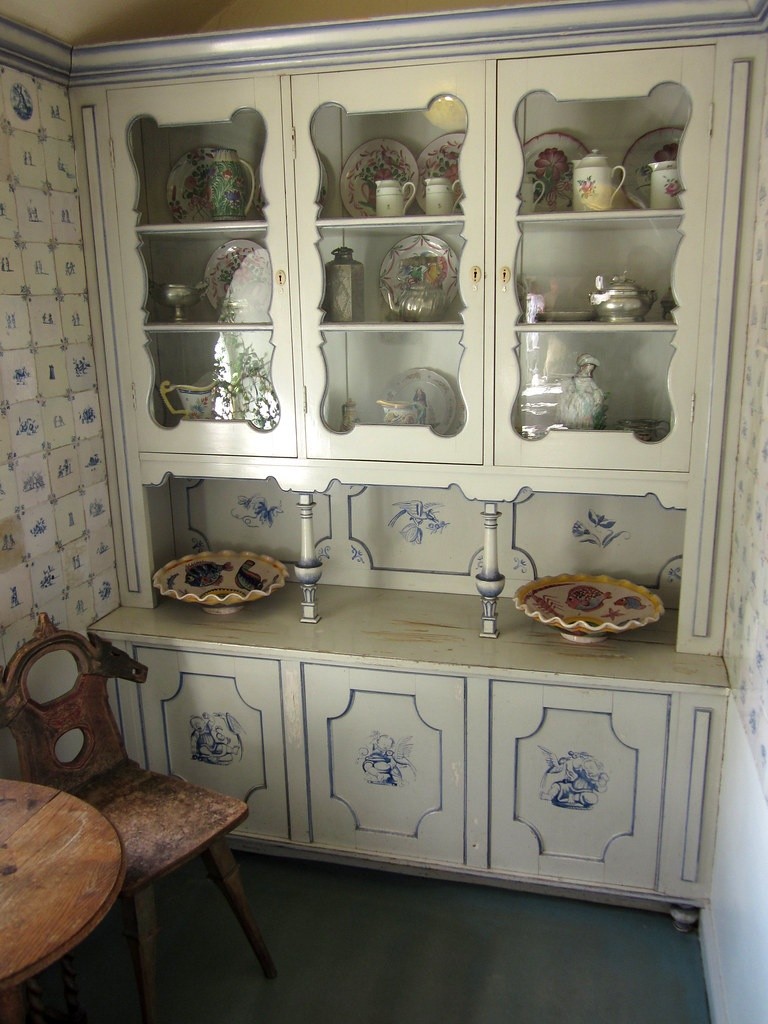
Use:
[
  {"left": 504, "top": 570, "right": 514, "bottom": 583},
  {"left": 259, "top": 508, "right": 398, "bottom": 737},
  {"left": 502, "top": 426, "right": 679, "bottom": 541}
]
[
  {"left": 289, "top": 58, "right": 489, "bottom": 470},
  {"left": 482, "top": 674, "right": 673, "bottom": 901},
  {"left": 106, "top": 77, "right": 298, "bottom": 461},
  {"left": 495, "top": 39, "right": 714, "bottom": 470},
  {"left": 297, "top": 659, "right": 471, "bottom": 873},
  {"left": 108, "top": 638, "right": 292, "bottom": 849}
]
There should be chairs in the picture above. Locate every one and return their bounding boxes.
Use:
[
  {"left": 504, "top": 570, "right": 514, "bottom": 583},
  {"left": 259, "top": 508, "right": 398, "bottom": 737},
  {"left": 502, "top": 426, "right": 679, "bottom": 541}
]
[{"left": 0, "top": 610, "right": 282, "bottom": 1024}]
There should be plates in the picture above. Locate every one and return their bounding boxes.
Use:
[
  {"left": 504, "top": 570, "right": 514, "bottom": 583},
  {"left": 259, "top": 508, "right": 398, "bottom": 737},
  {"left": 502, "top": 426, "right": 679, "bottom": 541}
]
[
  {"left": 254, "top": 149, "right": 329, "bottom": 217},
  {"left": 517, "top": 133, "right": 591, "bottom": 211},
  {"left": 340, "top": 139, "right": 419, "bottom": 219},
  {"left": 152, "top": 548, "right": 291, "bottom": 614},
  {"left": 413, "top": 133, "right": 469, "bottom": 214},
  {"left": 620, "top": 127, "right": 687, "bottom": 211},
  {"left": 386, "top": 368, "right": 456, "bottom": 435},
  {"left": 166, "top": 147, "right": 245, "bottom": 223},
  {"left": 513, "top": 574, "right": 666, "bottom": 645},
  {"left": 202, "top": 240, "right": 273, "bottom": 321},
  {"left": 378, "top": 234, "right": 460, "bottom": 319},
  {"left": 535, "top": 310, "right": 594, "bottom": 322}
]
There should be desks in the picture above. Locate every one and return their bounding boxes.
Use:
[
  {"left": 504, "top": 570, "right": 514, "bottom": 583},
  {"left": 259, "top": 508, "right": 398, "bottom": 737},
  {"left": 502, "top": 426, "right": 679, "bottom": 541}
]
[{"left": 0, "top": 778, "right": 128, "bottom": 1024}]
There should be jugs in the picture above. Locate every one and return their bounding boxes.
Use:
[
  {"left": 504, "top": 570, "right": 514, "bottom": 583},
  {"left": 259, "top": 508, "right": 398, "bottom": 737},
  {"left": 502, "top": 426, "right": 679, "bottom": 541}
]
[
  {"left": 374, "top": 180, "right": 417, "bottom": 217},
  {"left": 519, "top": 173, "right": 544, "bottom": 215},
  {"left": 209, "top": 148, "right": 255, "bottom": 221},
  {"left": 570, "top": 149, "right": 626, "bottom": 213},
  {"left": 375, "top": 400, "right": 424, "bottom": 427},
  {"left": 425, "top": 178, "right": 464, "bottom": 215},
  {"left": 160, "top": 369, "right": 220, "bottom": 421},
  {"left": 647, "top": 161, "right": 684, "bottom": 210}
]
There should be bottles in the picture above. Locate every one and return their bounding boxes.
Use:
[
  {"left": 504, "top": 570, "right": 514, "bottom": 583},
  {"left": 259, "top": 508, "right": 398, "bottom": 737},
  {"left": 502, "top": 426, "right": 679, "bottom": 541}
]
[
  {"left": 325, "top": 246, "right": 364, "bottom": 323},
  {"left": 340, "top": 400, "right": 360, "bottom": 432}
]
